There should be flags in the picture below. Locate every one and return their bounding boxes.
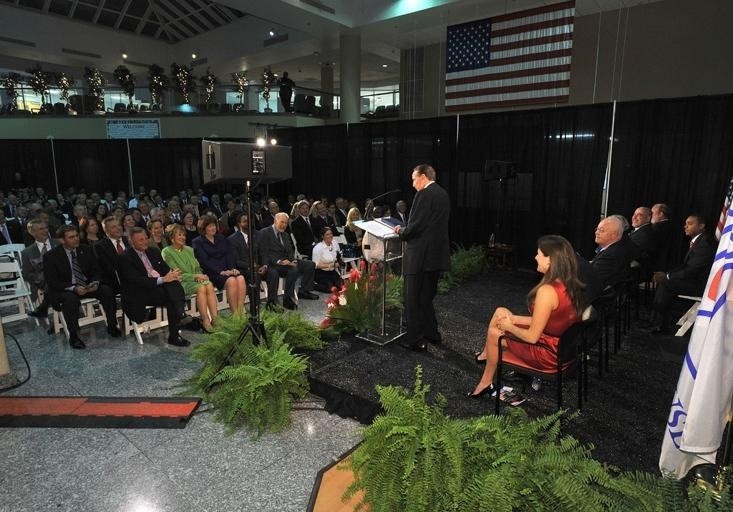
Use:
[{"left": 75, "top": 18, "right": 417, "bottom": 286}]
[
  {"left": 650, "top": 201, "right": 733, "bottom": 483},
  {"left": 712, "top": 181, "right": 733, "bottom": 241},
  {"left": 444, "top": 1, "right": 578, "bottom": 115}
]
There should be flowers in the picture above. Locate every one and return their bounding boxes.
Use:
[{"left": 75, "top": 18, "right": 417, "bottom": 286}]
[{"left": 314, "top": 256, "right": 403, "bottom": 336}]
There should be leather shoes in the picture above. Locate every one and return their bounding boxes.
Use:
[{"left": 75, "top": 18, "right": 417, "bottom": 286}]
[
  {"left": 397, "top": 333, "right": 441, "bottom": 352},
  {"left": 68, "top": 336, "right": 86, "bottom": 349},
  {"left": 27, "top": 308, "right": 48, "bottom": 318},
  {"left": 184, "top": 314, "right": 202, "bottom": 331},
  {"left": 297, "top": 291, "right": 319, "bottom": 300},
  {"left": 106, "top": 324, "right": 123, "bottom": 337},
  {"left": 168, "top": 335, "right": 190, "bottom": 346},
  {"left": 282, "top": 298, "right": 297, "bottom": 311},
  {"left": 268, "top": 299, "right": 284, "bottom": 314}
]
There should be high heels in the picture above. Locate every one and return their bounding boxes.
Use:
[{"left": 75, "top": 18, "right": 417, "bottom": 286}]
[
  {"left": 199, "top": 316, "right": 216, "bottom": 335},
  {"left": 467, "top": 382, "right": 495, "bottom": 399},
  {"left": 474, "top": 350, "right": 487, "bottom": 364}
]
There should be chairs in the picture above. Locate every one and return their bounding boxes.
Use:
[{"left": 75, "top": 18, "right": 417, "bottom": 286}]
[
  {"left": 1, "top": 94, "right": 403, "bottom": 118},
  {"left": 0, "top": 213, "right": 361, "bottom": 348},
  {"left": 490, "top": 240, "right": 705, "bottom": 421}
]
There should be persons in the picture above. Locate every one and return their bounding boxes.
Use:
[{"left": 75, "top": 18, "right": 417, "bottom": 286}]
[
  {"left": 160, "top": 223, "right": 219, "bottom": 333},
  {"left": 228, "top": 213, "right": 285, "bottom": 314},
  {"left": 116, "top": 227, "right": 190, "bottom": 346},
  {"left": 577, "top": 202, "right": 717, "bottom": 332},
  {"left": 312, "top": 227, "right": 344, "bottom": 293},
  {"left": 1, "top": 186, "right": 392, "bottom": 261},
  {"left": 22, "top": 219, "right": 61, "bottom": 335},
  {"left": 259, "top": 212, "right": 320, "bottom": 311},
  {"left": 191, "top": 215, "right": 246, "bottom": 313},
  {"left": 464, "top": 234, "right": 587, "bottom": 403},
  {"left": 392, "top": 163, "right": 453, "bottom": 355},
  {"left": 344, "top": 208, "right": 368, "bottom": 273},
  {"left": 276, "top": 71, "right": 297, "bottom": 113},
  {"left": 393, "top": 201, "right": 409, "bottom": 275},
  {"left": 43, "top": 225, "right": 122, "bottom": 349}
]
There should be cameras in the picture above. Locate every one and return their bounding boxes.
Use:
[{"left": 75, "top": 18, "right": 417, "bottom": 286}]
[{"left": 336, "top": 250, "right": 345, "bottom": 269}]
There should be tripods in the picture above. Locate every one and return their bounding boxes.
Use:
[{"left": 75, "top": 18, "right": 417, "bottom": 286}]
[{"left": 202, "top": 184, "right": 269, "bottom": 391}]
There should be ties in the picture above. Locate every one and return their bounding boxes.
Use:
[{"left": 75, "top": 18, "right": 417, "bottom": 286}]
[
  {"left": 144, "top": 217, "right": 149, "bottom": 223},
  {"left": 305, "top": 218, "right": 308, "bottom": 226},
  {"left": 69, "top": 251, "right": 89, "bottom": 289},
  {"left": 40, "top": 244, "right": 47, "bottom": 256},
  {"left": 11, "top": 206, "right": 16, "bottom": 217},
  {"left": 139, "top": 251, "right": 160, "bottom": 279},
  {"left": 216, "top": 204, "right": 222, "bottom": 215},
  {"left": 116, "top": 238, "right": 122, "bottom": 255},
  {"left": 276, "top": 232, "right": 281, "bottom": 244},
  {"left": 257, "top": 213, "right": 263, "bottom": 224},
  {"left": 175, "top": 214, "right": 178, "bottom": 221},
  {"left": 1, "top": 224, "right": 10, "bottom": 244}
]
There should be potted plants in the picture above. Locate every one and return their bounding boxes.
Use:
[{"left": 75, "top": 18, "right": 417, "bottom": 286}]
[
  {"left": 55, "top": 72, "right": 79, "bottom": 117},
  {"left": 258, "top": 65, "right": 275, "bottom": 117},
  {"left": 85, "top": 65, "right": 107, "bottom": 117}
]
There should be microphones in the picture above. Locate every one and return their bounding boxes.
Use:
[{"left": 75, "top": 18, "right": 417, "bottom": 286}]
[{"left": 363, "top": 188, "right": 401, "bottom": 222}]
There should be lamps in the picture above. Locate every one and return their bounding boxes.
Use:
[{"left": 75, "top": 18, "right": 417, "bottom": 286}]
[
  {"left": 190, "top": 47, "right": 200, "bottom": 59},
  {"left": 480, "top": 157, "right": 519, "bottom": 188},
  {"left": 198, "top": 138, "right": 296, "bottom": 190},
  {"left": 251, "top": 121, "right": 280, "bottom": 147}
]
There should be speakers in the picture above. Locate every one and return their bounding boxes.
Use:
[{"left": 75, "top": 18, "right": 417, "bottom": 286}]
[
  {"left": 484, "top": 159, "right": 518, "bottom": 182},
  {"left": 201, "top": 138, "right": 292, "bottom": 185}
]
